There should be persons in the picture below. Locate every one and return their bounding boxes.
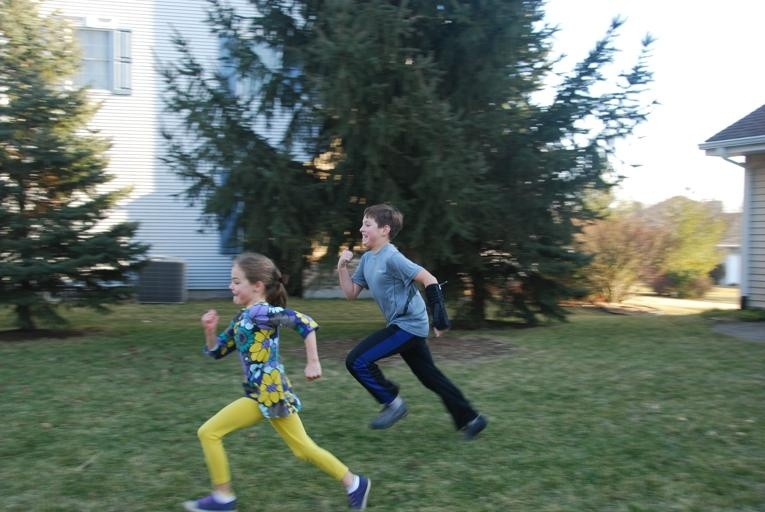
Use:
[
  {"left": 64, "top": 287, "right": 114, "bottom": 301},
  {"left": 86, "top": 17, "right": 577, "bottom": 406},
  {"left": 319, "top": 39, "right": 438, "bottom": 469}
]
[
  {"left": 337, "top": 203, "right": 490, "bottom": 445},
  {"left": 179, "top": 249, "right": 372, "bottom": 511}
]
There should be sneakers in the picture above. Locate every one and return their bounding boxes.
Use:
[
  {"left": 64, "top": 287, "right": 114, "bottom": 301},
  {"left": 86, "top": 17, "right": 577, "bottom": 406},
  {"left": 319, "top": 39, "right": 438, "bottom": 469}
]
[
  {"left": 457, "top": 414, "right": 487, "bottom": 439},
  {"left": 347, "top": 474, "right": 372, "bottom": 512},
  {"left": 370, "top": 400, "right": 408, "bottom": 430},
  {"left": 183, "top": 495, "right": 238, "bottom": 511}
]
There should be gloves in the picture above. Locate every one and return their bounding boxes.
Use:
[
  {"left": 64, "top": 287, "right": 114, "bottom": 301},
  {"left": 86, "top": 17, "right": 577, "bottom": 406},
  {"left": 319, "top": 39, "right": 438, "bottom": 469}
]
[{"left": 425, "top": 283, "right": 450, "bottom": 330}]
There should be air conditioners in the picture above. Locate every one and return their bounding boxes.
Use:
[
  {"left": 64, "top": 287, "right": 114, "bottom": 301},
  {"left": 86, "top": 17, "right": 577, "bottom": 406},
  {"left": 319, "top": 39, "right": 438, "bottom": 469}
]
[{"left": 137, "top": 260, "right": 186, "bottom": 305}]
